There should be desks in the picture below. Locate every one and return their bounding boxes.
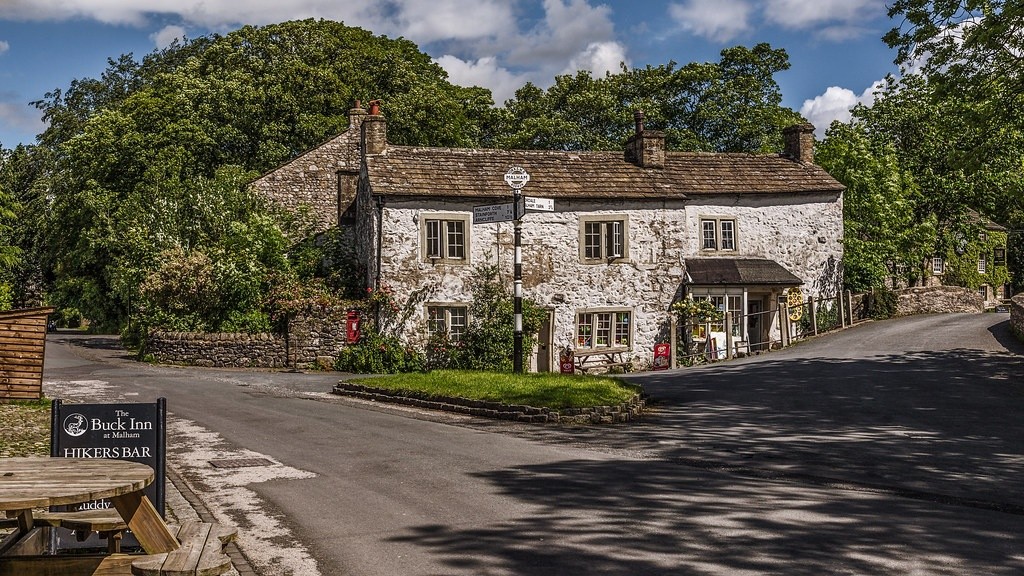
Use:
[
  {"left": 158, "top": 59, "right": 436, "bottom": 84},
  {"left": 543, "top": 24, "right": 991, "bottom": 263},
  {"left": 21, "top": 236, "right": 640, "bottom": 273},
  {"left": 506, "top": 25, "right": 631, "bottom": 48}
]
[
  {"left": 575, "top": 349, "right": 626, "bottom": 375},
  {"left": 0, "top": 457, "right": 182, "bottom": 576},
  {"left": 999, "top": 299, "right": 1011, "bottom": 306}
]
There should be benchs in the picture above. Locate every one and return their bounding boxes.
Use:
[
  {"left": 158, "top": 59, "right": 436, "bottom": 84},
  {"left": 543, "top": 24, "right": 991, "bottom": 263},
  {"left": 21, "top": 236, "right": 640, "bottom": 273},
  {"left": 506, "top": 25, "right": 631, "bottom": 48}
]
[
  {"left": 574, "top": 358, "right": 633, "bottom": 377},
  {"left": 132, "top": 520, "right": 238, "bottom": 576},
  {"left": 999, "top": 304, "right": 1011, "bottom": 313},
  {"left": 0, "top": 509, "right": 128, "bottom": 553}
]
